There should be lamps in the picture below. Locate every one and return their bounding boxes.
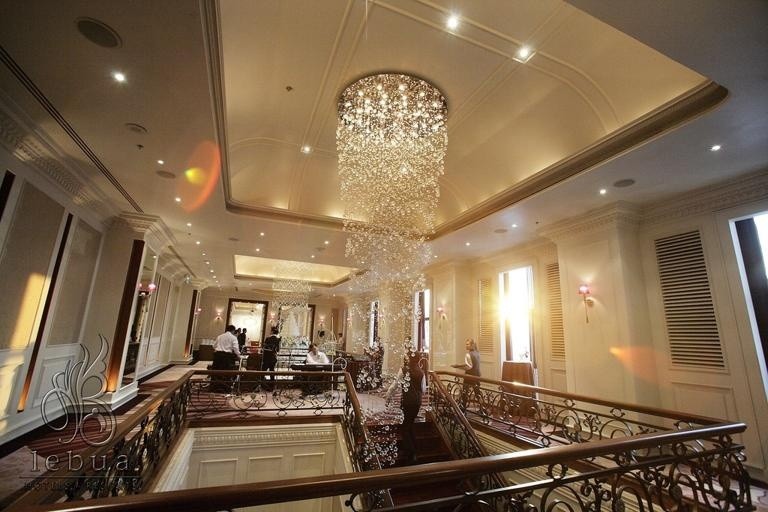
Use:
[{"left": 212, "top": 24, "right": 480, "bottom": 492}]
[{"left": 333, "top": 70, "right": 448, "bottom": 511}]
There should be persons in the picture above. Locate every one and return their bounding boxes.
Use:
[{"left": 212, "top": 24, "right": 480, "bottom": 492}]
[
  {"left": 261, "top": 326, "right": 282, "bottom": 381},
  {"left": 336, "top": 331, "right": 345, "bottom": 352},
  {"left": 384, "top": 347, "right": 428, "bottom": 466},
  {"left": 305, "top": 342, "right": 331, "bottom": 365},
  {"left": 450, "top": 337, "right": 490, "bottom": 418},
  {"left": 209, "top": 324, "right": 248, "bottom": 391}
]
